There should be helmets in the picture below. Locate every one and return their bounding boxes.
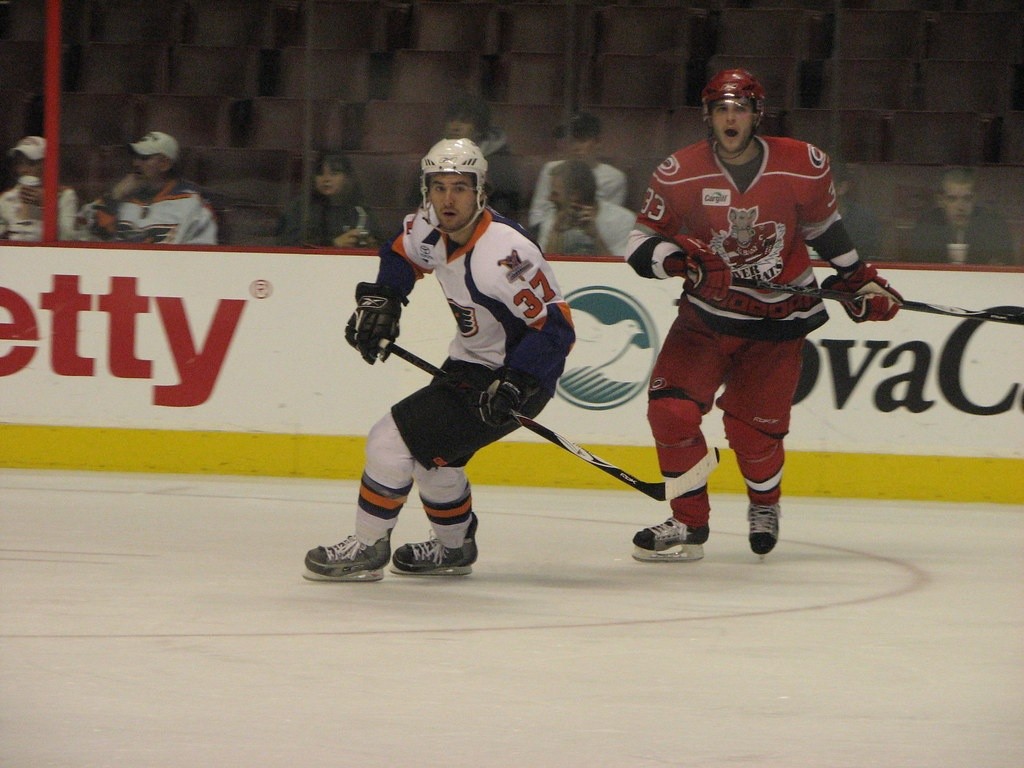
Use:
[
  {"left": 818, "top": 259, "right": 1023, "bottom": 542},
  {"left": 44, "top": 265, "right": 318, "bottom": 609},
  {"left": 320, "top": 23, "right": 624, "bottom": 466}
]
[
  {"left": 702, "top": 67, "right": 766, "bottom": 113},
  {"left": 421, "top": 138, "right": 488, "bottom": 194}
]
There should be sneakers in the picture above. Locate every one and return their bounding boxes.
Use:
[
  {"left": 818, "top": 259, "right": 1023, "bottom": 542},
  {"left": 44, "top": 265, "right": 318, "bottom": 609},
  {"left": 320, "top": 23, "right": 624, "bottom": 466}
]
[
  {"left": 302, "top": 527, "right": 392, "bottom": 582},
  {"left": 748, "top": 503, "right": 780, "bottom": 558},
  {"left": 389, "top": 512, "right": 478, "bottom": 575},
  {"left": 632, "top": 516, "right": 709, "bottom": 562}
]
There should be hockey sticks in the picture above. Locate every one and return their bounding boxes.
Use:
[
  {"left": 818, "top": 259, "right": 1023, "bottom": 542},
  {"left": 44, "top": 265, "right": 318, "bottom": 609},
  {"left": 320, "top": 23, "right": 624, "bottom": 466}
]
[
  {"left": 378, "top": 337, "right": 722, "bottom": 504},
  {"left": 728, "top": 273, "right": 1023, "bottom": 327}
]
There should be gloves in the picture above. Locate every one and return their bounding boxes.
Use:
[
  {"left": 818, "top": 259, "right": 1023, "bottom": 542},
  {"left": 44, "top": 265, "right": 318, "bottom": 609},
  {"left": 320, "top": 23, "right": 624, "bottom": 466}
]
[
  {"left": 475, "top": 365, "right": 540, "bottom": 427},
  {"left": 91, "top": 193, "right": 118, "bottom": 240},
  {"left": 821, "top": 257, "right": 904, "bottom": 323},
  {"left": 672, "top": 234, "right": 730, "bottom": 301},
  {"left": 344, "top": 282, "right": 409, "bottom": 365}
]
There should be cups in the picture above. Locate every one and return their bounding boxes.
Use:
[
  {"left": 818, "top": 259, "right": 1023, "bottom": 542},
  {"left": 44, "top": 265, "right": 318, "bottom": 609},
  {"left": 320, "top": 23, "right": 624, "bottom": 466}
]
[
  {"left": 17, "top": 175, "right": 40, "bottom": 204},
  {"left": 947, "top": 243, "right": 969, "bottom": 264}
]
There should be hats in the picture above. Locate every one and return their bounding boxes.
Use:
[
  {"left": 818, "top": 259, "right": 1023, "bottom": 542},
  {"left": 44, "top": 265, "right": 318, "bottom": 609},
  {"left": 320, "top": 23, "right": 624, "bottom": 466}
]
[
  {"left": 127, "top": 132, "right": 178, "bottom": 163},
  {"left": 9, "top": 136, "right": 46, "bottom": 160}
]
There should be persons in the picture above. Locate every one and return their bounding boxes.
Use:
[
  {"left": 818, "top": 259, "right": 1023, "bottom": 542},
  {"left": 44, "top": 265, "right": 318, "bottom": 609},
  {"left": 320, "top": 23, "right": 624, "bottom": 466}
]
[
  {"left": 527, "top": 111, "right": 638, "bottom": 256},
  {"left": 70, "top": 130, "right": 220, "bottom": 245},
  {"left": 304, "top": 137, "right": 577, "bottom": 576},
  {"left": 407, "top": 98, "right": 522, "bottom": 221},
  {"left": 900, "top": 166, "right": 1013, "bottom": 265},
  {"left": 278, "top": 150, "right": 385, "bottom": 247},
  {"left": 806, "top": 142, "right": 888, "bottom": 262},
  {"left": 0, "top": 137, "right": 79, "bottom": 242},
  {"left": 622, "top": 68, "right": 904, "bottom": 555}
]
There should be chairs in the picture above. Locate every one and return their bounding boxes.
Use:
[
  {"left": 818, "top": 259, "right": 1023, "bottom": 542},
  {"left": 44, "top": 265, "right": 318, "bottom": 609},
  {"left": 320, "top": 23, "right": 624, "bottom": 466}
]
[{"left": 0, "top": 0, "right": 1024, "bottom": 263}]
